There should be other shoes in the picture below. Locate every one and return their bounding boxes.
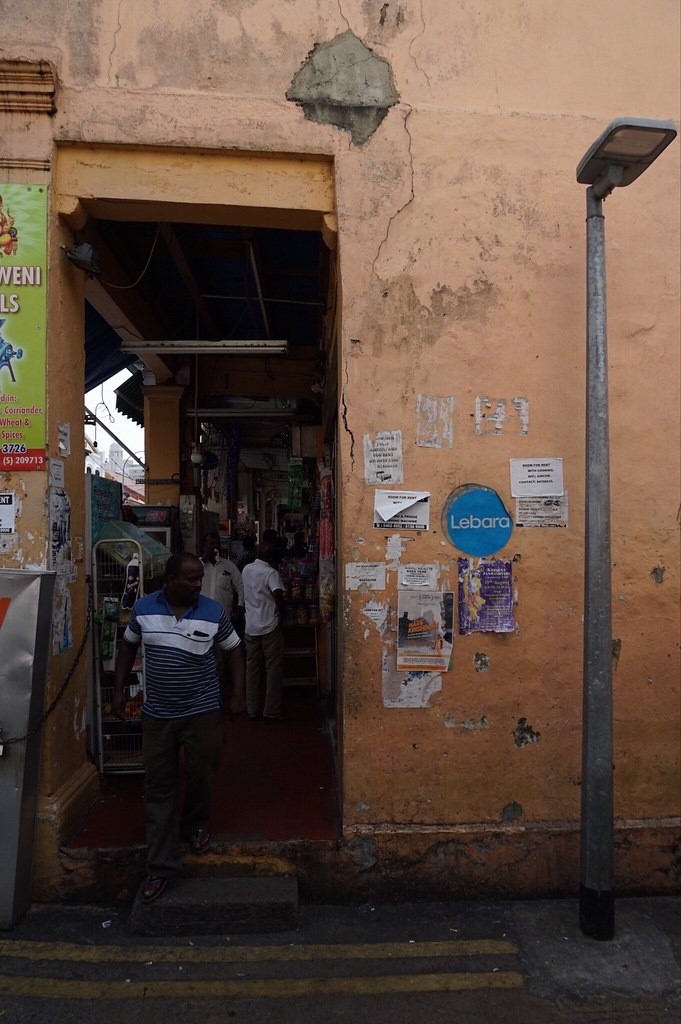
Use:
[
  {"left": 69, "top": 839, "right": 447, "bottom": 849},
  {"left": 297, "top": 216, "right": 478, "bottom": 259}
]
[
  {"left": 247, "top": 714, "right": 258, "bottom": 720},
  {"left": 264, "top": 713, "right": 287, "bottom": 725}
]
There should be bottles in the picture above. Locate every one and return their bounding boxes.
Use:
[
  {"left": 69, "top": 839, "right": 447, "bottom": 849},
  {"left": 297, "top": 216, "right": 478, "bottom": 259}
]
[{"left": 121, "top": 553, "right": 140, "bottom": 610}]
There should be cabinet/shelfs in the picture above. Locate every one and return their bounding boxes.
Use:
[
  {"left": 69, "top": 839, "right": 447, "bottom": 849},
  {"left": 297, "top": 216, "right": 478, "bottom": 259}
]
[{"left": 278, "top": 622, "right": 320, "bottom": 696}]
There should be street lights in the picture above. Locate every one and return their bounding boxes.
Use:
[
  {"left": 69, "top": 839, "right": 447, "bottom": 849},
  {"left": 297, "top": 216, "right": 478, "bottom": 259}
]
[{"left": 576, "top": 117, "right": 677, "bottom": 940}]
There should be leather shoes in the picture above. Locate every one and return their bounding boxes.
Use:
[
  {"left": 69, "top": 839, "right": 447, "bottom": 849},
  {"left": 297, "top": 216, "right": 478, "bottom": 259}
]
[
  {"left": 140, "top": 875, "right": 168, "bottom": 902},
  {"left": 190, "top": 820, "right": 211, "bottom": 853}
]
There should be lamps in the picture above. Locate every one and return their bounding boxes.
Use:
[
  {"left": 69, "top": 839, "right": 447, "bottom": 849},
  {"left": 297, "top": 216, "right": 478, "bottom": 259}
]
[
  {"left": 120, "top": 339, "right": 289, "bottom": 354},
  {"left": 189, "top": 355, "right": 203, "bottom": 465}
]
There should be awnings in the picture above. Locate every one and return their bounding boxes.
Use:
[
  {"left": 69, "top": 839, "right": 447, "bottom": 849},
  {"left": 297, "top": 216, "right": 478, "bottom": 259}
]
[
  {"left": 113, "top": 369, "right": 145, "bottom": 428},
  {"left": 84, "top": 298, "right": 149, "bottom": 472}
]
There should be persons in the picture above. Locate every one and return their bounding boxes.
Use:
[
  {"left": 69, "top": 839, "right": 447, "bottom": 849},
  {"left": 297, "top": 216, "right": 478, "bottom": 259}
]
[
  {"left": 240, "top": 529, "right": 288, "bottom": 724},
  {"left": 235, "top": 539, "right": 255, "bottom": 570},
  {"left": 197, "top": 533, "right": 243, "bottom": 684},
  {"left": 108, "top": 548, "right": 242, "bottom": 903}
]
